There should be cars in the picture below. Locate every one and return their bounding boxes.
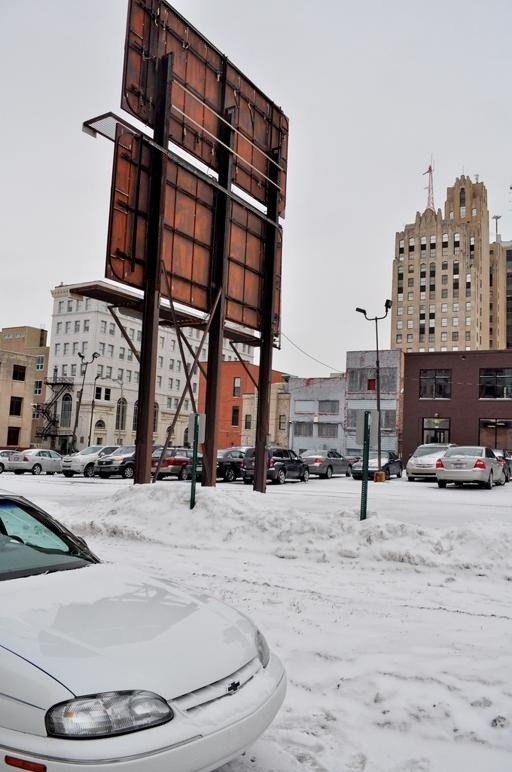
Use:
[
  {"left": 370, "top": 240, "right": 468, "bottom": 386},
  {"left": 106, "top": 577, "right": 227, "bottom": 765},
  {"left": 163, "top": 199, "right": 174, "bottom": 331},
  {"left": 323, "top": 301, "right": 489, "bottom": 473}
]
[{"left": 0, "top": 486, "right": 294, "bottom": 772}]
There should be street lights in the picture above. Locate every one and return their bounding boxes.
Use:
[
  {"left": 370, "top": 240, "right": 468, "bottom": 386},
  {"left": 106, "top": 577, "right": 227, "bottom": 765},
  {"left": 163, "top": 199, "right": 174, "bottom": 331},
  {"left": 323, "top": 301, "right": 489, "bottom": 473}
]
[
  {"left": 492, "top": 216, "right": 502, "bottom": 233},
  {"left": 68, "top": 350, "right": 100, "bottom": 452},
  {"left": 355, "top": 299, "right": 392, "bottom": 469},
  {"left": 105, "top": 374, "right": 123, "bottom": 443},
  {"left": 87, "top": 375, "right": 100, "bottom": 447}
]
